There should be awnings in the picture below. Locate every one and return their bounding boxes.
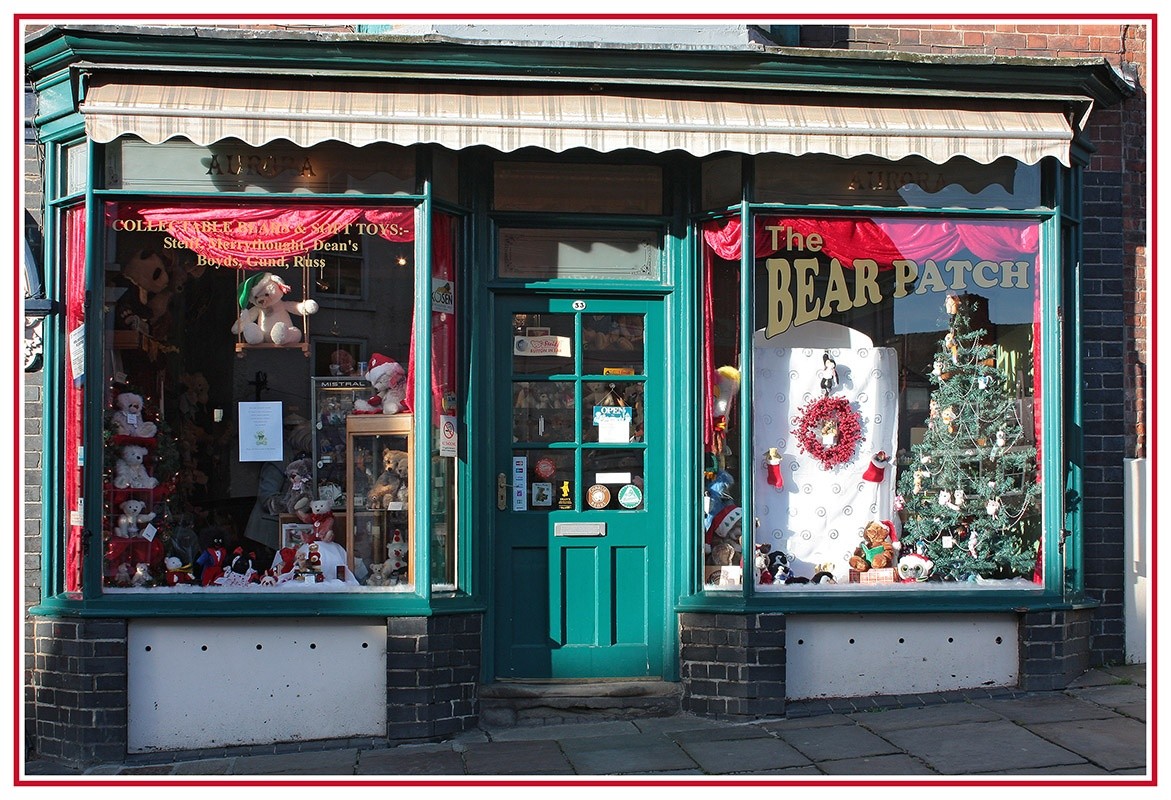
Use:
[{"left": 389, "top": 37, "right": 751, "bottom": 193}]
[{"left": 79, "top": 78, "right": 1084, "bottom": 171}]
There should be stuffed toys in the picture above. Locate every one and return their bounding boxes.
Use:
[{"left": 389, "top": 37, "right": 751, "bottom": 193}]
[
  {"left": 893, "top": 492, "right": 906, "bottom": 512},
  {"left": 816, "top": 353, "right": 840, "bottom": 395},
  {"left": 101, "top": 237, "right": 196, "bottom": 592},
  {"left": 515, "top": 382, "right": 645, "bottom": 443},
  {"left": 267, "top": 458, "right": 314, "bottom": 514},
  {"left": 897, "top": 552, "right": 934, "bottom": 584},
  {"left": 200, "top": 501, "right": 410, "bottom": 589},
  {"left": 709, "top": 363, "right": 741, "bottom": 454},
  {"left": 911, "top": 286, "right": 1020, "bottom": 572},
  {"left": 315, "top": 353, "right": 413, "bottom": 500},
  {"left": 228, "top": 270, "right": 318, "bottom": 346},
  {"left": 863, "top": 450, "right": 894, "bottom": 485},
  {"left": 848, "top": 518, "right": 901, "bottom": 572},
  {"left": 703, "top": 445, "right": 837, "bottom": 584}
]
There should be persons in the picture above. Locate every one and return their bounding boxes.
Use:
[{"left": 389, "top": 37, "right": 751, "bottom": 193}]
[{"left": 245, "top": 421, "right": 322, "bottom": 561}]
